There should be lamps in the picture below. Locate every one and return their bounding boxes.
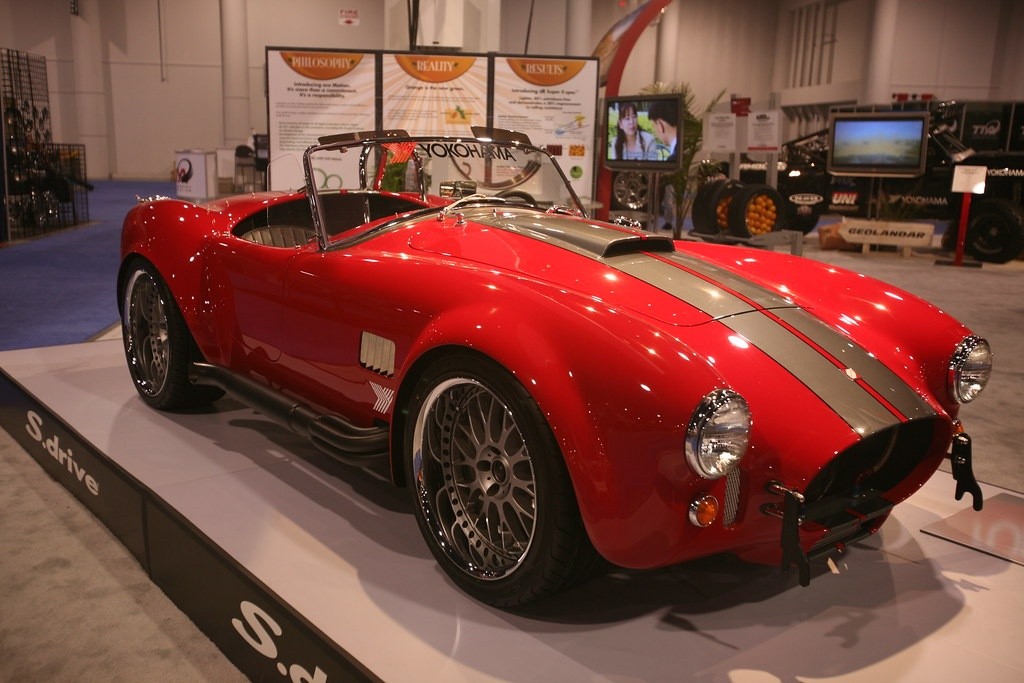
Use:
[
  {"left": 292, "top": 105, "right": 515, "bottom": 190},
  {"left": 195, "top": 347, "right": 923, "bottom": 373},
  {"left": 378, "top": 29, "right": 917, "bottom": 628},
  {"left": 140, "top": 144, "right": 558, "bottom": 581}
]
[{"left": 70, "top": 0, "right": 77, "bottom": 15}]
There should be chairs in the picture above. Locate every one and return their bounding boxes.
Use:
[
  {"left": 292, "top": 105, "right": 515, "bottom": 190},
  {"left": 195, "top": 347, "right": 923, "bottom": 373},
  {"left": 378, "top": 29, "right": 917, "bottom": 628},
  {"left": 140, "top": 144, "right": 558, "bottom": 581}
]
[
  {"left": 235, "top": 134, "right": 269, "bottom": 192},
  {"left": 241, "top": 225, "right": 315, "bottom": 248}
]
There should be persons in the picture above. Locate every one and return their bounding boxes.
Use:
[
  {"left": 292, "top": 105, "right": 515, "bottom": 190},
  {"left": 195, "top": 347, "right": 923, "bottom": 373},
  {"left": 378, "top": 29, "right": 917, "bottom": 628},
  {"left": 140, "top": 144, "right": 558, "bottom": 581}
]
[
  {"left": 648, "top": 100, "right": 678, "bottom": 161},
  {"left": 612, "top": 102, "right": 658, "bottom": 160}
]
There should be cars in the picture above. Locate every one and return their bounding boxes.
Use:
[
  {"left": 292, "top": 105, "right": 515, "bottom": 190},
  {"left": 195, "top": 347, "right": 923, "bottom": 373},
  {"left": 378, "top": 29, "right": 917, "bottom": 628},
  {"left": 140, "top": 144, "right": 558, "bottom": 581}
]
[{"left": 116, "top": 137, "right": 993, "bottom": 610}]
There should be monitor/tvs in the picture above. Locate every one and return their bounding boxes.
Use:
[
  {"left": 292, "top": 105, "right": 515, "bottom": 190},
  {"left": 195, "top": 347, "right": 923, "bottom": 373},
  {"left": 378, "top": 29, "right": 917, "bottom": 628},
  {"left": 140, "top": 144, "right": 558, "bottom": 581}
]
[
  {"left": 827, "top": 111, "right": 930, "bottom": 179},
  {"left": 605, "top": 92, "right": 684, "bottom": 170}
]
